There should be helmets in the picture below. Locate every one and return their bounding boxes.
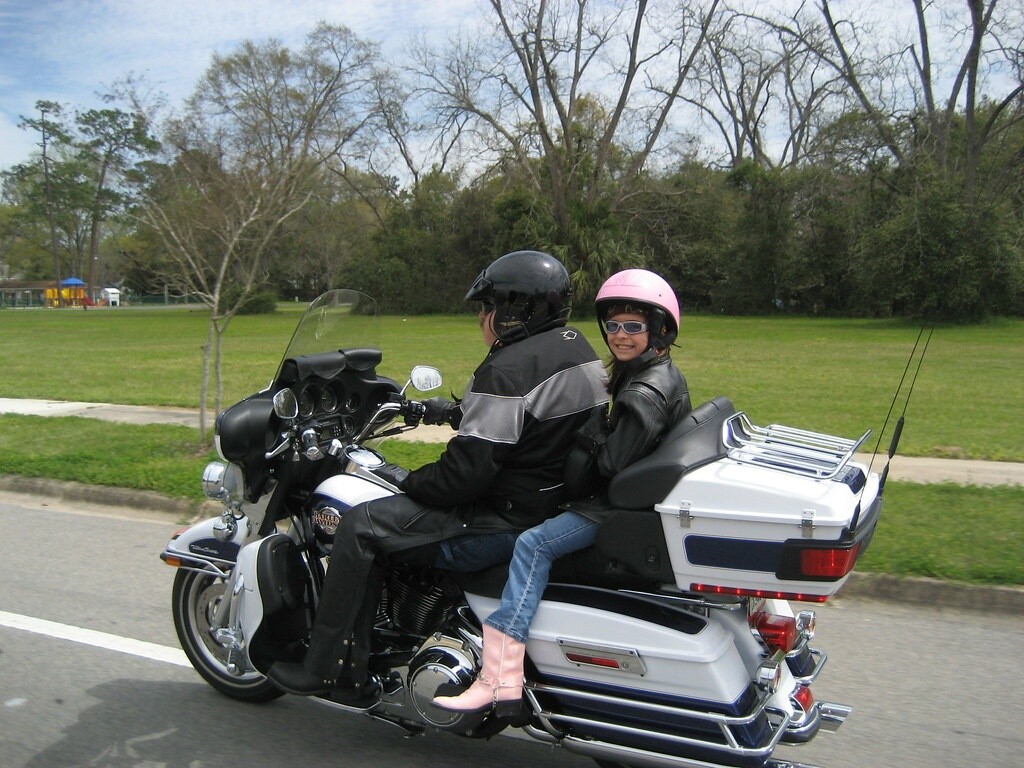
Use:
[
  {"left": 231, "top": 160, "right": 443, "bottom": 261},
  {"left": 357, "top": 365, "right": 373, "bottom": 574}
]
[
  {"left": 595, "top": 269, "right": 680, "bottom": 345},
  {"left": 463, "top": 250, "right": 571, "bottom": 342}
]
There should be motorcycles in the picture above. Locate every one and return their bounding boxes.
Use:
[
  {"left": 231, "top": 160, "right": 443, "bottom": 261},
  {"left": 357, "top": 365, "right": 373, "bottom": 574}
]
[{"left": 158, "top": 288, "right": 937, "bottom": 768}]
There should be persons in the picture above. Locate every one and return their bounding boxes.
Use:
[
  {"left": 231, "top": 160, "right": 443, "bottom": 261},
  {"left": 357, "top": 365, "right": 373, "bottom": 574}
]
[
  {"left": 261, "top": 250, "right": 611, "bottom": 701},
  {"left": 430, "top": 269, "right": 692, "bottom": 722}
]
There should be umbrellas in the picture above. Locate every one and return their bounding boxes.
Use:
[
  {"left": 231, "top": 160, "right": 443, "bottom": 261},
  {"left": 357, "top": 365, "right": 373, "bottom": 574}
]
[{"left": 61, "top": 277, "right": 86, "bottom": 299}]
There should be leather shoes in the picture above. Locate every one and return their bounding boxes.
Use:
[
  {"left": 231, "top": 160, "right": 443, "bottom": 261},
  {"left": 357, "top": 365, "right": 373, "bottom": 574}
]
[{"left": 267, "top": 660, "right": 380, "bottom": 706}]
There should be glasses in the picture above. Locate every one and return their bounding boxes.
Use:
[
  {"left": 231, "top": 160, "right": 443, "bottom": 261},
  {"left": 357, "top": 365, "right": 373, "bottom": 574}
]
[
  {"left": 480, "top": 300, "right": 495, "bottom": 315},
  {"left": 602, "top": 320, "right": 649, "bottom": 335}
]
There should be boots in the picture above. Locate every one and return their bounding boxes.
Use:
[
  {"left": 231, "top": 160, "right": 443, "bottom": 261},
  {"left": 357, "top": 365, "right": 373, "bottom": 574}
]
[{"left": 430, "top": 623, "right": 526, "bottom": 718}]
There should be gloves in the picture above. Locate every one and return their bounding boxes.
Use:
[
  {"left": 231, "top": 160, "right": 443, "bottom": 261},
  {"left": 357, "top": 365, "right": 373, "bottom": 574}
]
[{"left": 420, "top": 396, "right": 453, "bottom": 425}]
